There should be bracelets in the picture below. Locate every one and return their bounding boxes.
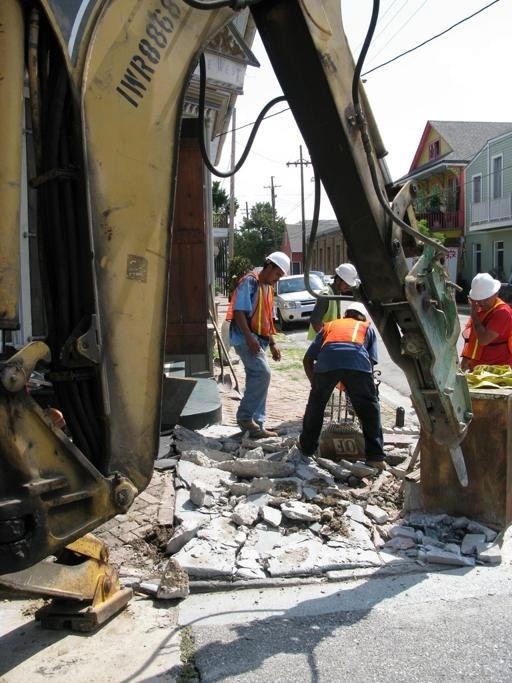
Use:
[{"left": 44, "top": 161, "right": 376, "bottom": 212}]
[{"left": 268, "top": 341, "right": 276, "bottom": 346}]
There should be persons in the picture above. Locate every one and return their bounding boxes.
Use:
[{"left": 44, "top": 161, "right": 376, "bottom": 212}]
[
  {"left": 293, "top": 300, "right": 386, "bottom": 470},
  {"left": 459, "top": 271, "right": 512, "bottom": 371},
  {"left": 305, "top": 261, "right": 360, "bottom": 340},
  {"left": 225, "top": 250, "right": 292, "bottom": 437}
]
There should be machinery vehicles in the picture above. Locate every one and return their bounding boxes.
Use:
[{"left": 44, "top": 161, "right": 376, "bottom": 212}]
[{"left": 1, "top": 0, "right": 512, "bottom": 634}]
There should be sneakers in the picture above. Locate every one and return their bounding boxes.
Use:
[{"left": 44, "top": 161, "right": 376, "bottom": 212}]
[
  {"left": 365, "top": 459, "right": 386, "bottom": 470},
  {"left": 238, "top": 417, "right": 259, "bottom": 431},
  {"left": 297, "top": 433, "right": 314, "bottom": 456},
  {"left": 250, "top": 429, "right": 278, "bottom": 438}
]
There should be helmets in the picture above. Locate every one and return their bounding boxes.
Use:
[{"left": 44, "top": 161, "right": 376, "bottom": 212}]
[
  {"left": 266, "top": 252, "right": 290, "bottom": 276},
  {"left": 335, "top": 263, "right": 358, "bottom": 287},
  {"left": 345, "top": 302, "right": 369, "bottom": 321},
  {"left": 469, "top": 273, "right": 502, "bottom": 301}
]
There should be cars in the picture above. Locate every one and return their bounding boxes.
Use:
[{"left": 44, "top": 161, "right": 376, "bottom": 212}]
[{"left": 270, "top": 269, "right": 362, "bottom": 329}]
